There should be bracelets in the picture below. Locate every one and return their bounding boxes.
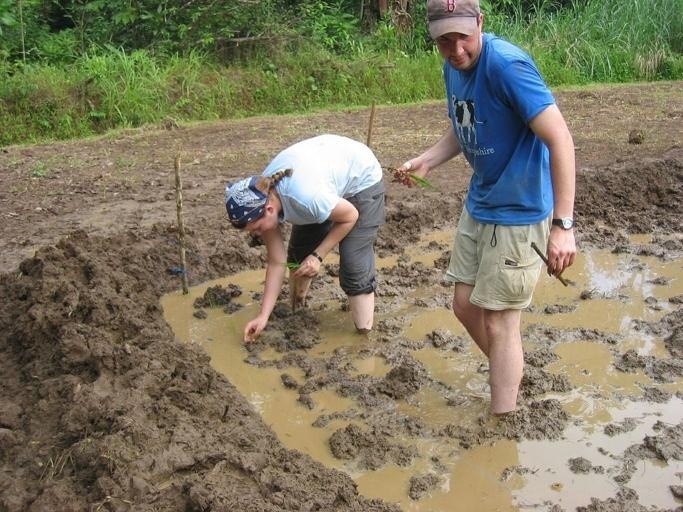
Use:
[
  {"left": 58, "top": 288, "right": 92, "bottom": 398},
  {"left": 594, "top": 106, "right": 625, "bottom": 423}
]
[{"left": 311, "top": 250, "right": 323, "bottom": 263}]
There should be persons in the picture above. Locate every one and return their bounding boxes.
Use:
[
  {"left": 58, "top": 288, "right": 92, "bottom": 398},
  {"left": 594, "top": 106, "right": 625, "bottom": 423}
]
[
  {"left": 394, "top": 0, "right": 578, "bottom": 415},
  {"left": 225, "top": 134, "right": 386, "bottom": 345}
]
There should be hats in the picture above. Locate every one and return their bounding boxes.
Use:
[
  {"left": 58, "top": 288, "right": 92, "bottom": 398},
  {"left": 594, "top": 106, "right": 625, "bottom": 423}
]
[{"left": 426, "top": 0, "right": 480, "bottom": 40}]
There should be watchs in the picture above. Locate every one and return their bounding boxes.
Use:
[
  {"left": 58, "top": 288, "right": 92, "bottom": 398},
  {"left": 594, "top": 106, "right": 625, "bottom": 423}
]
[{"left": 551, "top": 218, "right": 575, "bottom": 230}]
